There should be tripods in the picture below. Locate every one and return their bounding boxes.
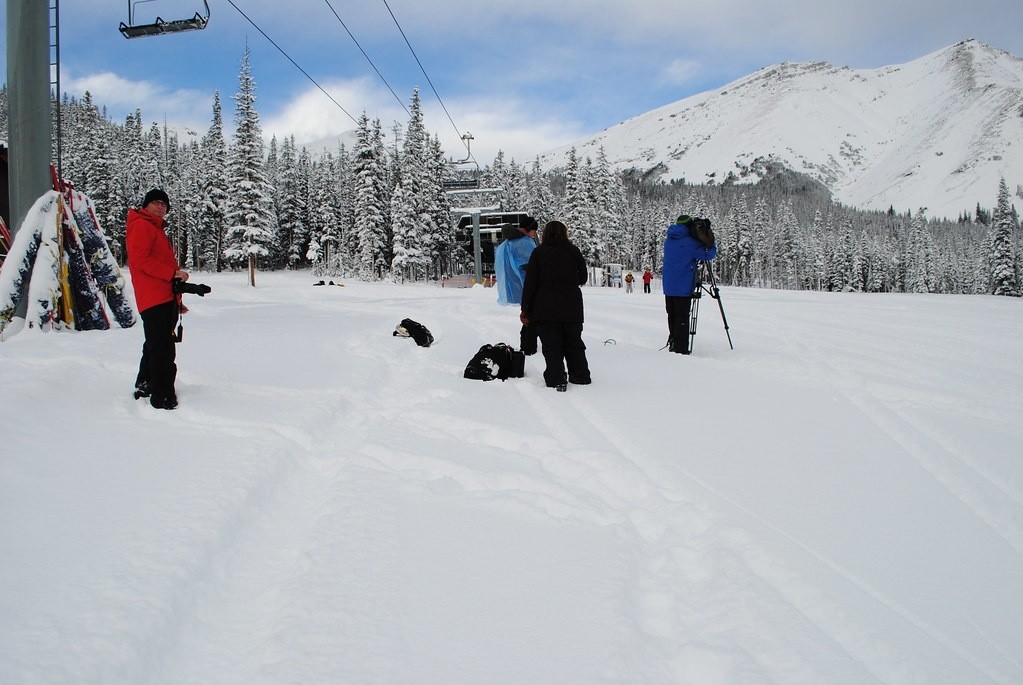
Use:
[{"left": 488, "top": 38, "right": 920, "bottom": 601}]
[{"left": 669, "top": 260, "right": 733, "bottom": 353}]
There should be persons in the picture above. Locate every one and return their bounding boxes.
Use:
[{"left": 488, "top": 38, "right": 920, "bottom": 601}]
[
  {"left": 521, "top": 221, "right": 592, "bottom": 392},
  {"left": 126, "top": 189, "right": 189, "bottom": 410},
  {"left": 643, "top": 270, "right": 654, "bottom": 293},
  {"left": 624, "top": 272, "right": 635, "bottom": 294},
  {"left": 494, "top": 216, "right": 538, "bottom": 306},
  {"left": 663, "top": 215, "right": 717, "bottom": 354}
]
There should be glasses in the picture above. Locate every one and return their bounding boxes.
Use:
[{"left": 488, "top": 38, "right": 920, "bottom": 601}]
[{"left": 151, "top": 200, "right": 167, "bottom": 208}]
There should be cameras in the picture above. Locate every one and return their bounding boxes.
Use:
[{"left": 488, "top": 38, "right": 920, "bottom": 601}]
[{"left": 171, "top": 277, "right": 211, "bottom": 297}]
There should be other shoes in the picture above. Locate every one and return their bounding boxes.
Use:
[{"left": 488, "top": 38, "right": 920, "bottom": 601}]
[{"left": 556, "top": 384, "right": 566, "bottom": 392}]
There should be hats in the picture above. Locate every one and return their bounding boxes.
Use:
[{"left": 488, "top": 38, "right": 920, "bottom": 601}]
[
  {"left": 519, "top": 217, "right": 538, "bottom": 231},
  {"left": 144, "top": 189, "right": 170, "bottom": 214},
  {"left": 677, "top": 215, "right": 692, "bottom": 226}
]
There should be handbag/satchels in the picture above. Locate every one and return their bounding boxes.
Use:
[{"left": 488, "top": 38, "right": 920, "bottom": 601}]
[
  {"left": 401, "top": 319, "right": 434, "bottom": 347},
  {"left": 464, "top": 343, "right": 526, "bottom": 380}
]
